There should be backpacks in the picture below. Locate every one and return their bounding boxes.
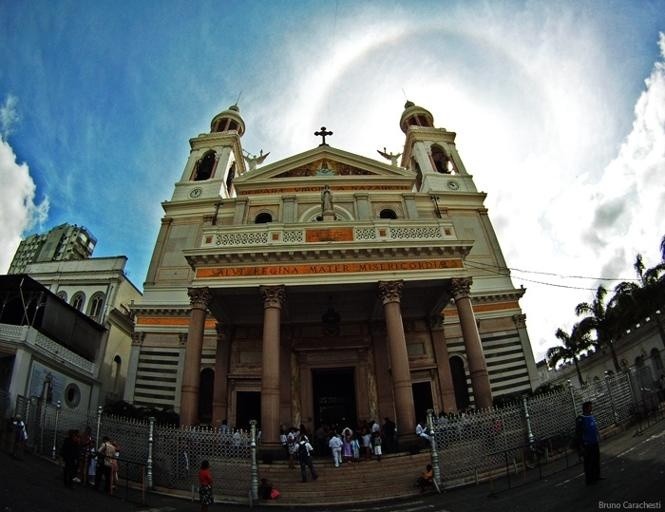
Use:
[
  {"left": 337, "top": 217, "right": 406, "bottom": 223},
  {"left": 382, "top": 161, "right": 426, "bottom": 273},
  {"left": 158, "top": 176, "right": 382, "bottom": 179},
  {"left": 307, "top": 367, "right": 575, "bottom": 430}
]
[{"left": 297, "top": 442, "right": 309, "bottom": 460}]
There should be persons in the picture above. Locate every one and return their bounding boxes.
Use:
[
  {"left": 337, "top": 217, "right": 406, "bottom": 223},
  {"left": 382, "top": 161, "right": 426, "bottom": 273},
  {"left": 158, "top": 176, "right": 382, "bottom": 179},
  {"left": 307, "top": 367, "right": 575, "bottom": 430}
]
[
  {"left": 11, "top": 413, "right": 28, "bottom": 459},
  {"left": 233, "top": 428, "right": 244, "bottom": 456},
  {"left": 416, "top": 408, "right": 477, "bottom": 448},
  {"left": 61, "top": 425, "right": 120, "bottom": 494},
  {"left": 412, "top": 464, "right": 434, "bottom": 492},
  {"left": 180, "top": 440, "right": 190, "bottom": 488},
  {"left": 218, "top": 419, "right": 232, "bottom": 458},
  {"left": 575, "top": 400, "right": 606, "bottom": 489},
  {"left": 279, "top": 415, "right": 398, "bottom": 481},
  {"left": 197, "top": 459, "right": 214, "bottom": 512}
]
